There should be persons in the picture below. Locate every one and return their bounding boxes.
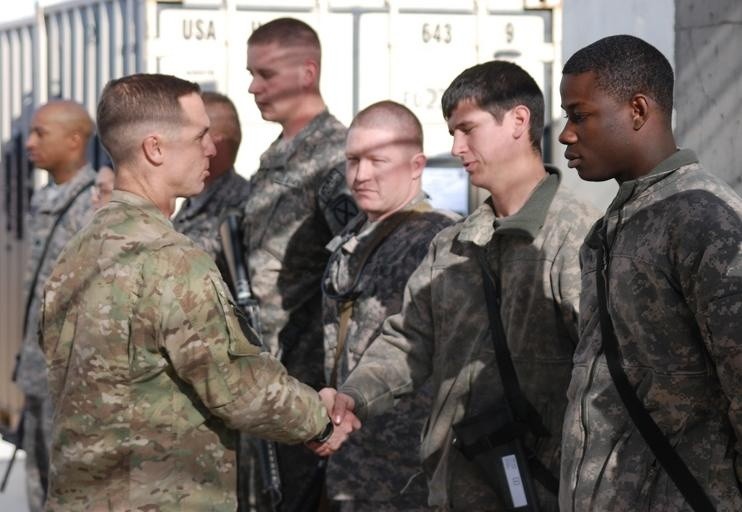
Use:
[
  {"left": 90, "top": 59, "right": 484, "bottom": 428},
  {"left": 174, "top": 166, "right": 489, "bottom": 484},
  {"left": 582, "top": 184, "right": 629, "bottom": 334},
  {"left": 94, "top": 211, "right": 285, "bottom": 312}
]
[
  {"left": 172, "top": 90, "right": 251, "bottom": 265},
  {"left": 305, "top": 60, "right": 601, "bottom": 511},
  {"left": 90, "top": 162, "right": 115, "bottom": 212},
  {"left": 34, "top": 74, "right": 362, "bottom": 511},
  {"left": 11, "top": 99, "right": 100, "bottom": 512},
  {"left": 558, "top": 34, "right": 741, "bottom": 510},
  {"left": 195, "top": 16, "right": 361, "bottom": 511},
  {"left": 319, "top": 99, "right": 469, "bottom": 512}
]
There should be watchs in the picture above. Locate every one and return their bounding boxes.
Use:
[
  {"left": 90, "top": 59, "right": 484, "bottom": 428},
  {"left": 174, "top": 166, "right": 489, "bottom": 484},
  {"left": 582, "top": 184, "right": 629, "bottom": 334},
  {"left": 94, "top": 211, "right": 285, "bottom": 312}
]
[{"left": 309, "top": 412, "right": 334, "bottom": 444}]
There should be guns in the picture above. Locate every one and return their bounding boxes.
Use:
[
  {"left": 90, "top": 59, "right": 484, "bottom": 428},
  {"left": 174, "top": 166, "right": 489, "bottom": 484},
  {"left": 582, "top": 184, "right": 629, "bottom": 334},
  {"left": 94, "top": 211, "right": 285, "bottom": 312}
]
[{"left": 216, "top": 205, "right": 283, "bottom": 512}]
